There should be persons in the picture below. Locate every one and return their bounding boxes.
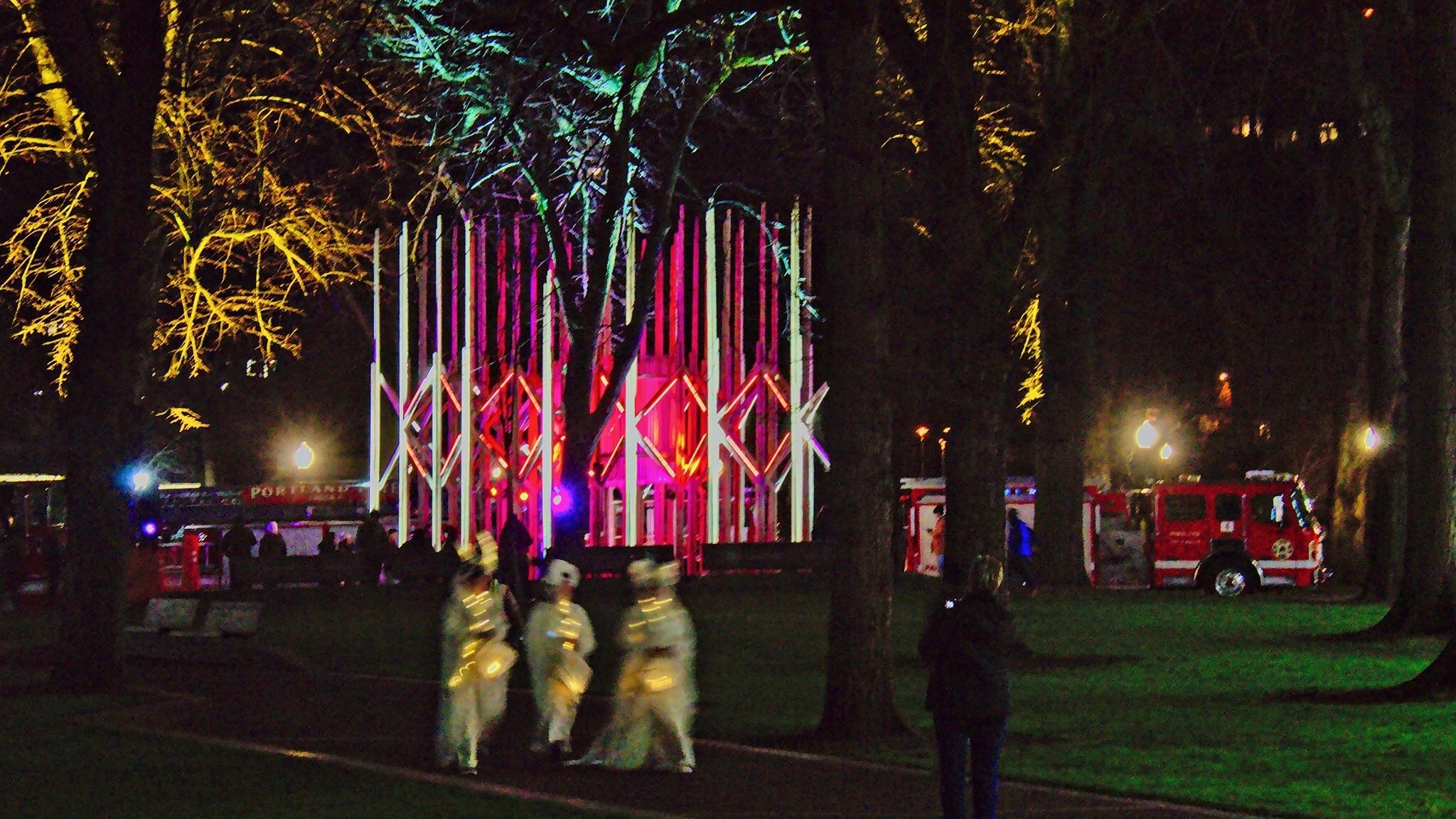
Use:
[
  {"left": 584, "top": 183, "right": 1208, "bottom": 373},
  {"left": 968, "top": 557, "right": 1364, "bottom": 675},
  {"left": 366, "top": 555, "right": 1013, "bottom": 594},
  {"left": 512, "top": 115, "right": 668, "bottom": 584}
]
[
  {"left": 1130, "top": 496, "right": 1151, "bottom": 535},
  {"left": 927, "top": 504, "right": 946, "bottom": 572},
  {"left": 918, "top": 555, "right": 1021, "bottom": 818},
  {"left": 40, "top": 527, "right": 60, "bottom": 602},
  {"left": 220, "top": 510, "right": 441, "bottom": 595},
  {"left": 443, "top": 532, "right": 702, "bottom": 774},
  {"left": 1006, "top": 508, "right": 1040, "bottom": 598}
]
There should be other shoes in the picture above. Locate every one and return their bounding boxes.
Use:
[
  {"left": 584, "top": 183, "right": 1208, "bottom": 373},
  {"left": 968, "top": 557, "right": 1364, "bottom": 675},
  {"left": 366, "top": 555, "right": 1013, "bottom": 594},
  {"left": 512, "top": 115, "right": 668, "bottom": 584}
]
[
  {"left": 671, "top": 765, "right": 692, "bottom": 773},
  {"left": 463, "top": 766, "right": 477, "bottom": 775}
]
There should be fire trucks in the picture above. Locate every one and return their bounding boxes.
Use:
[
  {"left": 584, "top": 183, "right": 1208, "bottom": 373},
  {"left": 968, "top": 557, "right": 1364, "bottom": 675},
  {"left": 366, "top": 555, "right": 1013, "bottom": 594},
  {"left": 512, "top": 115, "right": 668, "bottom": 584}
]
[{"left": 903, "top": 468, "right": 1331, "bottom": 601}]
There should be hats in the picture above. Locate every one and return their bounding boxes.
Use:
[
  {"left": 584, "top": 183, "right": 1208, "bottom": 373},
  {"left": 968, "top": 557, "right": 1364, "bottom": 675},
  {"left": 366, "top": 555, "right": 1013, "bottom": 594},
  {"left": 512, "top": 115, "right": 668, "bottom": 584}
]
[{"left": 541, "top": 558, "right": 582, "bottom": 587}]
